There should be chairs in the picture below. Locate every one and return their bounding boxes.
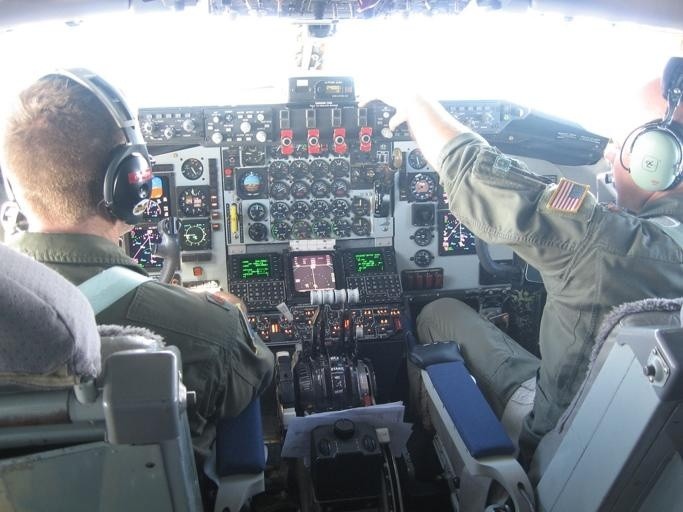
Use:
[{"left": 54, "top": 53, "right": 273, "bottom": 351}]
[
  {"left": 0, "top": 241, "right": 270, "bottom": 512},
  {"left": 408, "top": 296, "right": 683, "bottom": 512}
]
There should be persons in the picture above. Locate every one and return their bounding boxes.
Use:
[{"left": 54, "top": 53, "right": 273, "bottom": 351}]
[
  {"left": 0, "top": 70, "right": 276, "bottom": 496},
  {"left": 346, "top": 75, "right": 683, "bottom": 475}
]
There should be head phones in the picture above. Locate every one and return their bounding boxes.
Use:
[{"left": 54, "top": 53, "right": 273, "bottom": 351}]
[
  {"left": 39, "top": 64, "right": 153, "bottom": 225},
  {"left": 628, "top": 56, "right": 683, "bottom": 192}
]
